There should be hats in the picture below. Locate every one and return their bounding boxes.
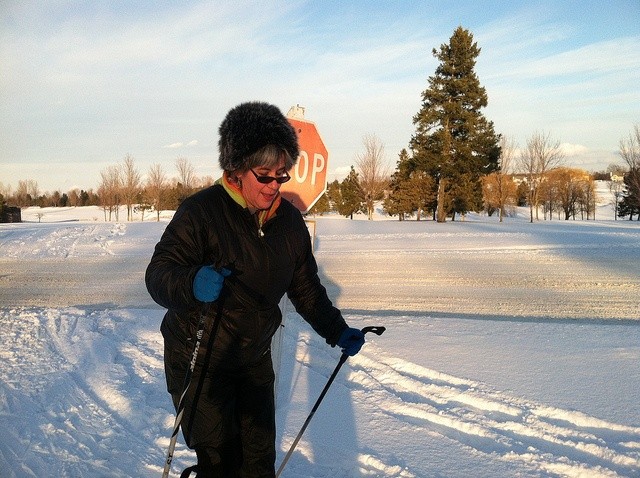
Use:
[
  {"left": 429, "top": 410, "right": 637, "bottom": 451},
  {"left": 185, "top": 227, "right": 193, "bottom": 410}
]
[{"left": 217, "top": 100, "right": 299, "bottom": 171}]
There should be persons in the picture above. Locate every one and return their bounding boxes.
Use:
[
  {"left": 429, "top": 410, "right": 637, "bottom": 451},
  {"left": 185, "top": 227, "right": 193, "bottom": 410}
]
[{"left": 145, "top": 102, "right": 365, "bottom": 478}]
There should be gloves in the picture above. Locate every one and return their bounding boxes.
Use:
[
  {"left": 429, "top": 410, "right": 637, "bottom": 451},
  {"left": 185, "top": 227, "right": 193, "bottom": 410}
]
[
  {"left": 337, "top": 328, "right": 365, "bottom": 357},
  {"left": 193, "top": 263, "right": 231, "bottom": 304}
]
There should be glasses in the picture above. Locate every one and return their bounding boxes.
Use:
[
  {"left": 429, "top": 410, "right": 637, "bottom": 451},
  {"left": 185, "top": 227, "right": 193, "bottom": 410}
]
[{"left": 249, "top": 168, "right": 291, "bottom": 184}]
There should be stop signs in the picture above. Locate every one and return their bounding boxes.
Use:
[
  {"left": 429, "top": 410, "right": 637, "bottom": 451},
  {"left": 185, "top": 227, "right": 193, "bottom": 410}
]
[{"left": 279, "top": 116, "right": 328, "bottom": 214}]
